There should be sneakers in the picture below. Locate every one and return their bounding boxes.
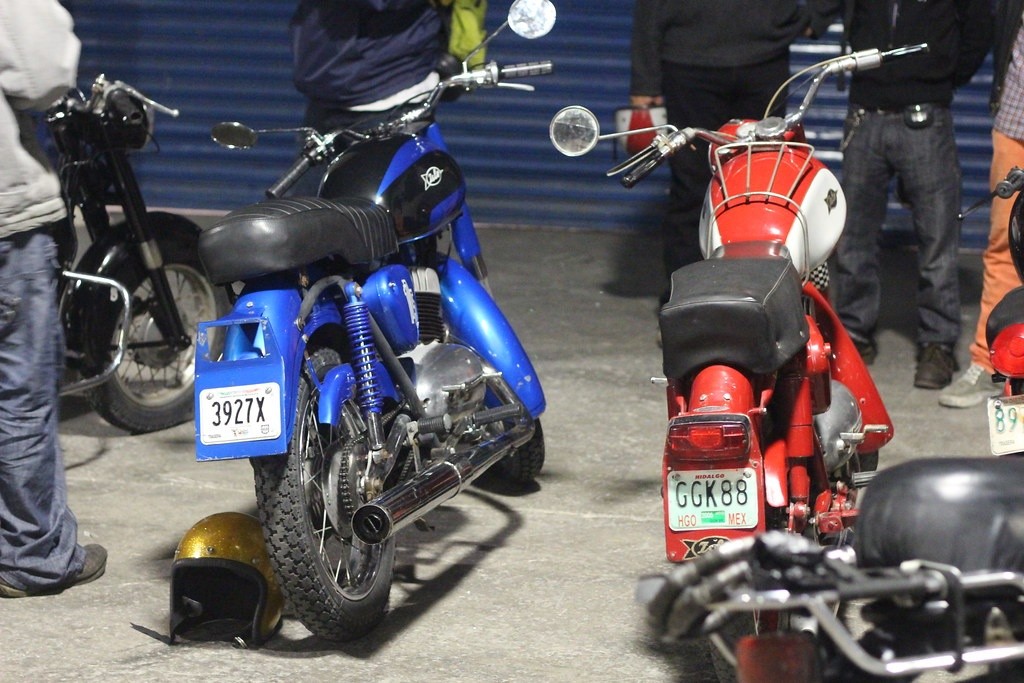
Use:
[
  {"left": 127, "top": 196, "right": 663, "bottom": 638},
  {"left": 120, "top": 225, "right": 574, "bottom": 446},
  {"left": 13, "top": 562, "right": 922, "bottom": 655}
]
[{"left": 938, "top": 365, "right": 1004, "bottom": 407}]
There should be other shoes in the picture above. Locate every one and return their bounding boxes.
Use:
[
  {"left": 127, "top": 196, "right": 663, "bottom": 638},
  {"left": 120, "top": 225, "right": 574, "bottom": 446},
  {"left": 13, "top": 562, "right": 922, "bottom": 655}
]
[
  {"left": 914, "top": 343, "right": 953, "bottom": 388},
  {"left": 0, "top": 544, "right": 107, "bottom": 598},
  {"left": 856, "top": 345, "right": 880, "bottom": 365}
]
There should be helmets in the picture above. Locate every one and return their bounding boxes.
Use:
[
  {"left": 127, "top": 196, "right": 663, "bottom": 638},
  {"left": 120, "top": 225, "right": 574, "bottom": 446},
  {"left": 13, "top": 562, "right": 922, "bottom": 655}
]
[
  {"left": 170, "top": 513, "right": 284, "bottom": 645},
  {"left": 613, "top": 105, "right": 667, "bottom": 156}
]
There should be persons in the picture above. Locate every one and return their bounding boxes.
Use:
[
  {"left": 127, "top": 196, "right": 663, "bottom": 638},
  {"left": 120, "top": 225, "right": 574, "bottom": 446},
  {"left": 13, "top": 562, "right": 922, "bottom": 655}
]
[
  {"left": 629, "top": 0, "right": 1024, "bottom": 407},
  {"left": 0, "top": 0, "right": 108, "bottom": 597},
  {"left": 293, "top": 0, "right": 487, "bottom": 198}
]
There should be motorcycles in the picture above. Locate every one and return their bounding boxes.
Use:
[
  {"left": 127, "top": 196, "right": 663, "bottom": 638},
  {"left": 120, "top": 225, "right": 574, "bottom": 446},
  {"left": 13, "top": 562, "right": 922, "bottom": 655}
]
[
  {"left": 45, "top": 75, "right": 237, "bottom": 432},
  {"left": 195, "top": 0, "right": 558, "bottom": 638},
  {"left": 639, "top": 168, "right": 1024, "bottom": 682},
  {"left": 549, "top": 44, "right": 927, "bottom": 683}
]
